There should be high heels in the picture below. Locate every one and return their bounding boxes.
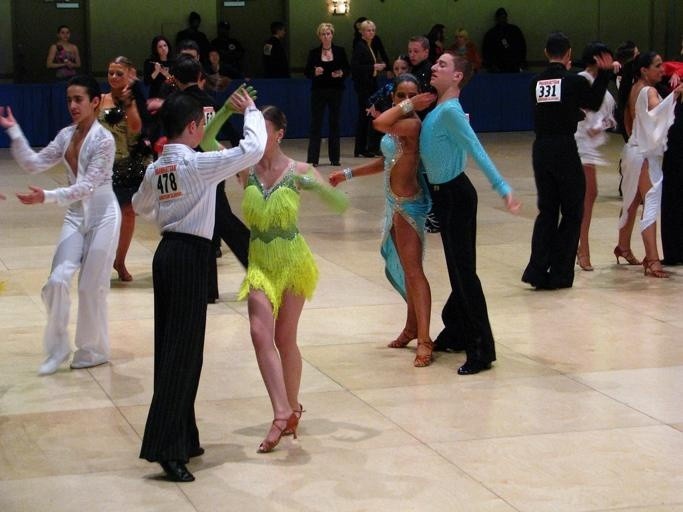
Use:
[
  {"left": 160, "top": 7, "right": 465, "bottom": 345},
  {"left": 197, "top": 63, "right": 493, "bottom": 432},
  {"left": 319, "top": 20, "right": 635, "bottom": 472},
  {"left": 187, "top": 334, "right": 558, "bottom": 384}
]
[
  {"left": 390, "top": 328, "right": 417, "bottom": 348},
  {"left": 642, "top": 255, "right": 669, "bottom": 277},
  {"left": 576, "top": 246, "right": 593, "bottom": 271},
  {"left": 355, "top": 151, "right": 375, "bottom": 157},
  {"left": 113, "top": 260, "right": 133, "bottom": 281},
  {"left": 330, "top": 159, "right": 341, "bottom": 166},
  {"left": 414, "top": 342, "right": 434, "bottom": 366},
  {"left": 614, "top": 246, "right": 642, "bottom": 265},
  {"left": 258, "top": 403, "right": 306, "bottom": 451}
]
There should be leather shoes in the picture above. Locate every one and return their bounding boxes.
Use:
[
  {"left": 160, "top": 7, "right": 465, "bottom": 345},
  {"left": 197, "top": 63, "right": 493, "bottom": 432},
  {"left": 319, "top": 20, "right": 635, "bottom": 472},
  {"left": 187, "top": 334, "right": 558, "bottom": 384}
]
[
  {"left": 158, "top": 460, "right": 194, "bottom": 482},
  {"left": 458, "top": 362, "right": 490, "bottom": 374}
]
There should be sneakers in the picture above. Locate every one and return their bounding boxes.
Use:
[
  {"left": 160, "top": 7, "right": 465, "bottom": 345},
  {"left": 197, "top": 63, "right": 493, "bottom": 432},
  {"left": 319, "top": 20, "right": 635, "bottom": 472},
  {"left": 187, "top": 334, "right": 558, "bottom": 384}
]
[
  {"left": 41, "top": 355, "right": 66, "bottom": 373},
  {"left": 70, "top": 360, "right": 96, "bottom": 368}
]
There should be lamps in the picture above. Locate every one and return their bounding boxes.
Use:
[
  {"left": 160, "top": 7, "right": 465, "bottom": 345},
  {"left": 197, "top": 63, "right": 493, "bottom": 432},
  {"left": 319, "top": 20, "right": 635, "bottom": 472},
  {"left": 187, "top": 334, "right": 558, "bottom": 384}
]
[{"left": 328, "top": 1, "right": 349, "bottom": 16}]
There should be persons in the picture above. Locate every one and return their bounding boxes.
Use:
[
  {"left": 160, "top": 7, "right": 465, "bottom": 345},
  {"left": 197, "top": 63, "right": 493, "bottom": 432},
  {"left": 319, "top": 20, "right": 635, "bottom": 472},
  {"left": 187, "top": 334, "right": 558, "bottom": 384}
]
[
  {"left": 197, "top": 78, "right": 350, "bottom": 454},
  {"left": 521, "top": 32, "right": 681, "bottom": 290},
  {"left": 42, "top": 11, "right": 286, "bottom": 378},
  {"left": 129, "top": 85, "right": 268, "bottom": 484},
  {"left": 0, "top": 72, "right": 125, "bottom": 375},
  {"left": 303, "top": 9, "right": 526, "bottom": 376}
]
